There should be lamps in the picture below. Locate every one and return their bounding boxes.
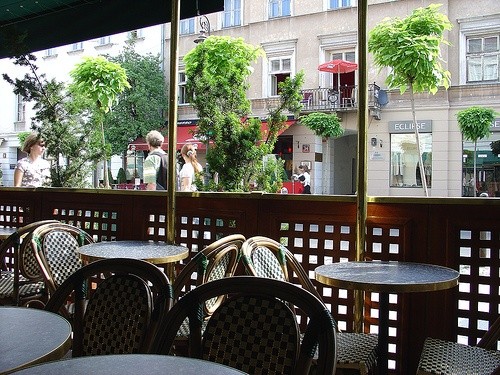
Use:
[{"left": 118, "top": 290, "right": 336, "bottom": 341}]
[
  {"left": 3, "top": 153, "right": 6, "bottom": 158},
  {"left": 371, "top": 138, "right": 376, "bottom": 146}
]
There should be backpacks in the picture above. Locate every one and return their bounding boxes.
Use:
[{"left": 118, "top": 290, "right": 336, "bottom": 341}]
[{"left": 150, "top": 152, "right": 168, "bottom": 189}]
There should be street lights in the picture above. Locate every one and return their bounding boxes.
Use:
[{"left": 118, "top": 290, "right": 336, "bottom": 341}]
[{"left": 193, "top": 16, "right": 211, "bottom": 188}]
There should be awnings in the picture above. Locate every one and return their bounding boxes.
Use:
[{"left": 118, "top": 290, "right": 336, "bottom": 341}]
[
  {"left": 417, "top": 151, "right": 500, "bottom": 169},
  {"left": 128, "top": 120, "right": 295, "bottom": 151}
]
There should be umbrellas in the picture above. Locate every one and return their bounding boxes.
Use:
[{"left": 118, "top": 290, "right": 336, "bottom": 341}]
[{"left": 318, "top": 59, "right": 357, "bottom": 107}]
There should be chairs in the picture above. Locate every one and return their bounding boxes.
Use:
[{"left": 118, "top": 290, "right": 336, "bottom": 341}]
[
  {"left": 342, "top": 88, "right": 356, "bottom": 107},
  {"left": 299, "top": 92, "right": 313, "bottom": 110},
  {"left": 0, "top": 219, "right": 500, "bottom": 375},
  {"left": 317, "top": 92, "right": 328, "bottom": 108}
]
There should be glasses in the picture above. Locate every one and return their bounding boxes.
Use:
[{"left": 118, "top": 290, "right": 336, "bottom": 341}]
[{"left": 36, "top": 144, "right": 45, "bottom": 147}]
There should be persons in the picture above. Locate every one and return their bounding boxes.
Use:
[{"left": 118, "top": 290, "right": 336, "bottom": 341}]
[
  {"left": 298, "top": 165, "right": 312, "bottom": 194},
  {"left": 142, "top": 129, "right": 168, "bottom": 191},
  {"left": 13, "top": 134, "right": 53, "bottom": 241},
  {"left": 393, "top": 150, "right": 406, "bottom": 185},
  {"left": 462, "top": 173, "right": 475, "bottom": 196},
  {"left": 179, "top": 144, "right": 205, "bottom": 192}
]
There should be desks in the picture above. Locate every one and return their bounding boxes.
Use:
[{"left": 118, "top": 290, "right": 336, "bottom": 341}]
[
  {"left": 314, "top": 261, "right": 460, "bottom": 375},
  {"left": 276, "top": 181, "right": 305, "bottom": 194},
  {"left": 74, "top": 241, "right": 189, "bottom": 308},
  {"left": 0, "top": 228, "right": 29, "bottom": 272},
  {"left": 0, "top": 306, "right": 73, "bottom": 375},
  {"left": 1, "top": 354, "right": 250, "bottom": 375}
]
